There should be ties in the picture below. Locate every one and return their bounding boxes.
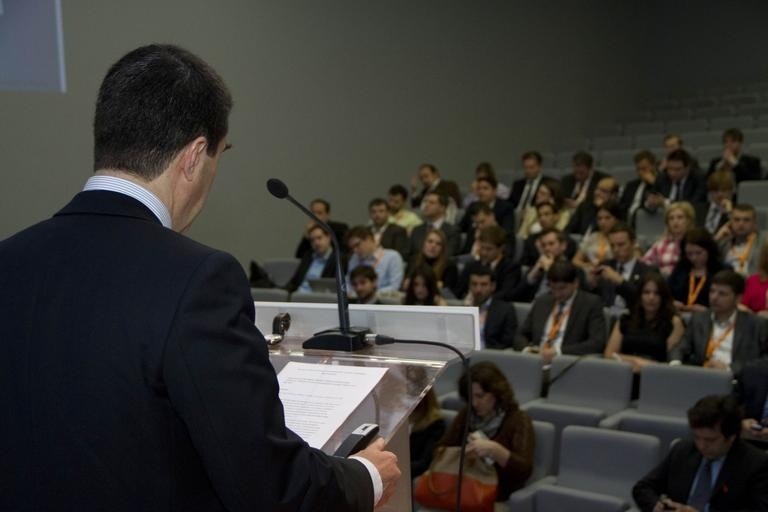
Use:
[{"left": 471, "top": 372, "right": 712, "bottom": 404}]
[
  {"left": 619, "top": 262, "right": 623, "bottom": 273},
  {"left": 574, "top": 180, "right": 585, "bottom": 200},
  {"left": 486, "top": 260, "right": 491, "bottom": 269},
  {"left": 546, "top": 302, "right": 565, "bottom": 349},
  {"left": 675, "top": 179, "right": 681, "bottom": 202},
  {"left": 522, "top": 181, "right": 533, "bottom": 210},
  {"left": 687, "top": 455, "right": 716, "bottom": 512},
  {"left": 710, "top": 206, "right": 719, "bottom": 230}
]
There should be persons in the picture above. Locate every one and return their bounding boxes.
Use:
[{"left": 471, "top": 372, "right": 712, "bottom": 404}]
[
  {"left": 285, "top": 225, "right": 340, "bottom": 292},
  {"left": 506, "top": 151, "right": 545, "bottom": 211},
  {"left": 409, "top": 163, "right": 463, "bottom": 211},
  {"left": 385, "top": 183, "right": 422, "bottom": 238},
  {"left": 619, "top": 151, "right": 663, "bottom": 227},
  {"left": 514, "top": 228, "right": 587, "bottom": 304},
  {"left": 572, "top": 200, "right": 629, "bottom": 298},
  {"left": 562, "top": 176, "right": 635, "bottom": 240},
  {"left": 453, "top": 226, "right": 522, "bottom": 301},
  {"left": 411, "top": 190, "right": 460, "bottom": 259},
  {"left": 631, "top": 396, "right": 768, "bottom": 511},
  {"left": 407, "top": 388, "right": 445, "bottom": 480},
  {"left": 518, "top": 176, "right": 573, "bottom": 240},
  {"left": 603, "top": 274, "right": 683, "bottom": 368},
  {"left": 704, "top": 127, "right": 763, "bottom": 182},
  {"left": 713, "top": 204, "right": 768, "bottom": 283},
  {"left": 517, "top": 202, "right": 580, "bottom": 268},
  {"left": 667, "top": 269, "right": 768, "bottom": 371},
  {"left": 652, "top": 148, "right": 710, "bottom": 229},
  {"left": 346, "top": 264, "right": 382, "bottom": 304},
  {"left": 657, "top": 133, "right": 685, "bottom": 173},
  {"left": 0, "top": 44, "right": 406, "bottom": 511},
  {"left": 294, "top": 198, "right": 351, "bottom": 259},
  {"left": 459, "top": 176, "right": 516, "bottom": 232},
  {"left": 343, "top": 225, "right": 405, "bottom": 294},
  {"left": 401, "top": 229, "right": 458, "bottom": 295},
  {"left": 513, "top": 262, "right": 607, "bottom": 364},
  {"left": 666, "top": 227, "right": 733, "bottom": 312},
  {"left": 559, "top": 151, "right": 611, "bottom": 208},
  {"left": 641, "top": 202, "right": 699, "bottom": 279},
  {"left": 433, "top": 362, "right": 537, "bottom": 512},
  {"left": 693, "top": 171, "right": 739, "bottom": 236},
  {"left": 401, "top": 265, "right": 447, "bottom": 307},
  {"left": 460, "top": 202, "right": 516, "bottom": 263},
  {"left": 586, "top": 223, "right": 660, "bottom": 308},
  {"left": 466, "top": 268, "right": 518, "bottom": 350},
  {"left": 365, "top": 198, "right": 411, "bottom": 262}
]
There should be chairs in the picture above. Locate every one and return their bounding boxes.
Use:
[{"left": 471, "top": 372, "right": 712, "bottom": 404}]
[
  {"left": 263, "top": 257, "right": 299, "bottom": 285},
  {"left": 437, "top": 345, "right": 546, "bottom": 410},
  {"left": 550, "top": 84, "right": 765, "bottom": 178},
  {"left": 599, "top": 365, "right": 734, "bottom": 429},
  {"left": 513, "top": 425, "right": 665, "bottom": 511},
  {"left": 520, "top": 356, "right": 632, "bottom": 426},
  {"left": 635, "top": 206, "right": 668, "bottom": 248},
  {"left": 513, "top": 421, "right": 557, "bottom": 476},
  {"left": 246, "top": 285, "right": 286, "bottom": 303},
  {"left": 732, "top": 178, "right": 768, "bottom": 212},
  {"left": 293, "top": 291, "right": 338, "bottom": 304}
]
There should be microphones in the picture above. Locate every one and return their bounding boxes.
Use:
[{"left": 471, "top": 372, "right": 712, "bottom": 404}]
[{"left": 265, "top": 177, "right": 351, "bottom": 329}]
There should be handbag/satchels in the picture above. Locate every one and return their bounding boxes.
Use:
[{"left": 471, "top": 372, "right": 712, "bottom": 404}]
[{"left": 414, "top": 447, "right": 497, "bottom": 511}]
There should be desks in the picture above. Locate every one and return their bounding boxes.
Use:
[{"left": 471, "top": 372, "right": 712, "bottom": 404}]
[{"left": 258, "top": 334, "right": 475, "bottom": 511}]
